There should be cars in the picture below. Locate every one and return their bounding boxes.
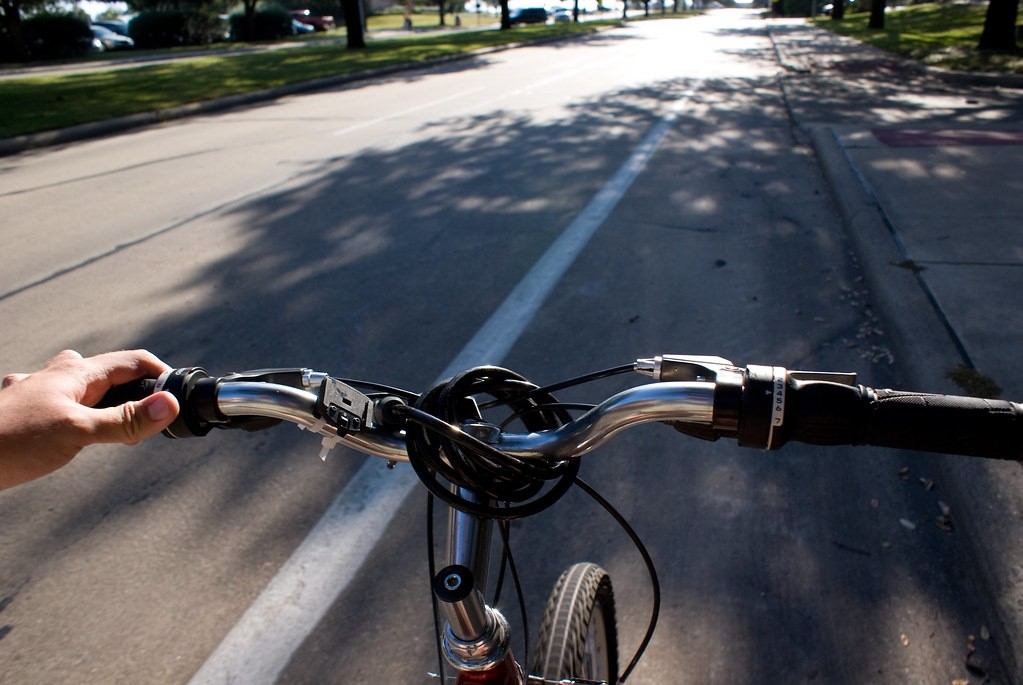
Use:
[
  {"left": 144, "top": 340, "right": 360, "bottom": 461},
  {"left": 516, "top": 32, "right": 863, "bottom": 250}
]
[
  {"left": 507, "top": 7, "right": 548, "bottom": 26},
  {"left": 91, "top": 19, "right": 131, "bottom": 36},
  {"left": 555, "top": 13, "right": 571, "bottom": 24},
  {"left": 295, "top": 15, "right": 336, "bottom": 32},
  {"left": 823, "top": 3, "right": 833, "bottom": 17},
  {"left": 89, "top": 26, "right": 134, "bottom": 50},
  {"left": 291, "top": 19, "right": 316, "bottom": 35},
  {"left": 89, "top": 37, "right": 106, "bottom": 55}
]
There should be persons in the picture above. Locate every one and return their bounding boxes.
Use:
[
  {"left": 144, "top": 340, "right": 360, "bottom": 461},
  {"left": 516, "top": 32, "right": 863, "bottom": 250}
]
[{"left": 0, "top": 348, "right": 180, "bottom": 493}]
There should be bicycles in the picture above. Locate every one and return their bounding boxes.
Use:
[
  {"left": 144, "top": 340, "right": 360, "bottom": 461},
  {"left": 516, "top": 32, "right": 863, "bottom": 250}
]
[{"left": 90, "top": 350, "right": 1022, "bottom": 685}]
